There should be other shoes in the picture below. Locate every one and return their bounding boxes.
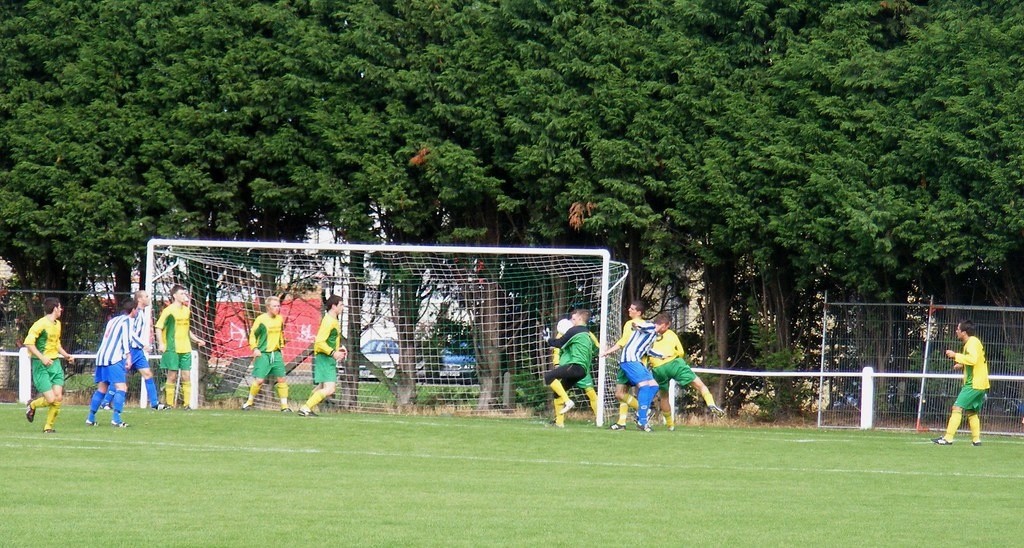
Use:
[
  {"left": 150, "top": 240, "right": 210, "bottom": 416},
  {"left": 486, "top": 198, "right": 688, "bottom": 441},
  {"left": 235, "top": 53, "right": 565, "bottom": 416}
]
[
  {"left": 559, "top": 399, "right": 574, "bottom": 414},
  {"left": 86, "top": 421, "right": 98, "bottom": 426},
  {"left": 544, "top": 423, "right": 564, "bottom": 429},
  {"left": 610, "top": 423, "right": 626, "bottom": 431},
  {"left": 111, "top": 421, "right": 128, "bottom": 428},
  {"left": 298, "top": 409, "right": 318, "bottom": 417},
  {"left": 100, "top": 404, "right": 111, "bottom": 410},
  {"left": 242, "top": 402, "right": 255, "bottom": 410},
  {"left": 281, "top": 408, "right": 291, "bottom": 413},
  {"left": 663, "top": 411, "right": 674, "bottom": 431},
  {"left": 636, "top": 424, "right": 652, "bottom": 432},
  {"left": 184, "top": 406, "right": 191, "bottom": 410},
  {"left": 152, "top": 402, "right": 170, "bottom": 411},
  {"left": 708, "top": 405, "right": 725, "bottom": 417},
  {"left": 972, "top": 441, "right": 981, "bottom": 446},
  {"left": 931, "top": 436, "right": 953, "bottom": 445}
]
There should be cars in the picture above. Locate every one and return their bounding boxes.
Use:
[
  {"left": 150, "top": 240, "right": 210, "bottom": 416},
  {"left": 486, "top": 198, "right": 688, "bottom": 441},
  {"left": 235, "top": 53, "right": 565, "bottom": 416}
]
[{"left": 338, "top": 339, "right": 482, "bottom": 382}]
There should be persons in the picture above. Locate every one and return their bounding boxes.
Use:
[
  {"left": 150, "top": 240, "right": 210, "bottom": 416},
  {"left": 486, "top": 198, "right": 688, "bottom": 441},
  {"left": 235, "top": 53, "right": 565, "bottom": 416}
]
[
  {"left": 540, "top": 308, "right": 599, "bottom": 427},
  {"left": 100, "top": 289, "right": 166, "bottom": 411},
  {"left": 85, "top": 297, "right": 139, "bottom": 428},
  {"left": 296, "top": 295, "right": 347, "bottom": 417},
  {"left": 241, "top": 296, "right": 293, "bottom": 412},
  {"left": 642, "top": 326, "right": 724, "bottom": 431},
  {"left": 930, "top": 320, "right": 991, "bottom": 447},
  {"left": 619, "top": 314, "right": 668, "bottom": 432},
  {"left": 599, "top": 300, "right": 652, "bottom": 430},
  {"left": 154, "top": 284, "right": 207, "bottom": 410},
  {"left": 23, "top": 297, "right": 74, "bottom": 434}
]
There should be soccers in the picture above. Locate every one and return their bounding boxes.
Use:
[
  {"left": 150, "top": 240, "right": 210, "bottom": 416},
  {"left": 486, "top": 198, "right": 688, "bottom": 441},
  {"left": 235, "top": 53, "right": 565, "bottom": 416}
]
[{"left": 556, "top": 318, "right": 574, "bottom": 336}]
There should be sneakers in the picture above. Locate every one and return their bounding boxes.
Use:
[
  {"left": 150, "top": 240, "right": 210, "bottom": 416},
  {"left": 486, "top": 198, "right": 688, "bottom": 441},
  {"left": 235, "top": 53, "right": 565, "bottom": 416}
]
[
  {"left": 26, "top": 400, "right": 35, "bottom": 422},
  {"left": 45, "top": 429, "right": 55, "bottom": 433}
]
